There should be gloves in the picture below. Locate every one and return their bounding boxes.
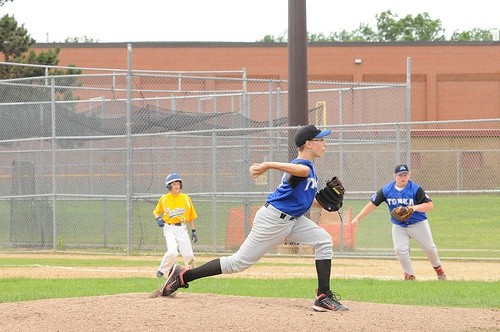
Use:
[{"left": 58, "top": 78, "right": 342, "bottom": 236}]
[
  {"left": 191, "top": 229, "right": 198, "bottom": 243},
  {"left": 156, "top": 217, "right": 165, "bottom": 227}
]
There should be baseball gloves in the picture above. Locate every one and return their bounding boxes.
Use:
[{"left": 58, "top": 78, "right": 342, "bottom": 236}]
[
  {"left": 315, "top": 177, "right": 347, "bottom": 213},
  {"left": 391, "top": 205, "right": 414, "bottom": 221}
]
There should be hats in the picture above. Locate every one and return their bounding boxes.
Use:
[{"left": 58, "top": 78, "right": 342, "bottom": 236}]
[
  {"left": 395, "top": 165, "right": 409, "bottom": 174},
  {"left": 294, "top": 125, "right": 331, "bottom": 147}
]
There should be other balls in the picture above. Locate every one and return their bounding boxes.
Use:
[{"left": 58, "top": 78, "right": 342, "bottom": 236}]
[{"left": 250, "top": 164, "right": 260, "bottom": 177}]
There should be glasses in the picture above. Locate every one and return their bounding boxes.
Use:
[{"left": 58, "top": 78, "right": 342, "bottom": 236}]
[{"left": 311, "top": 138, "right": 324, "bottom": 141}]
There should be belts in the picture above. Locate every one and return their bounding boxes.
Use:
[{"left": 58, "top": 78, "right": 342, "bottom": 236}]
[
  {"left": 264, "top": 203, "right": 294, "bottom": 221},
  {"left": 168, "top": 223, "right": 181, "bottom": 226}
]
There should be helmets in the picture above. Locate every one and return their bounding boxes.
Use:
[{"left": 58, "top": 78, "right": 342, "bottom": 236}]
[{"left": 166, "top": 174, "right": 181, "bottom": 185}]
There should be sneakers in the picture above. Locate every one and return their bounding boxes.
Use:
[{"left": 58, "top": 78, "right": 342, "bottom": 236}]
[
  {"left": 156, "top": 270, "right": 163, "bottom": 278},
  {"left": 438, "top": 273, "right": 446, "bottom": 280},
  {"left": 313, "top": 289, "right": 349, "bottom": 312},
  {"left": 160, "top": 263, "right": 189, "bottom": 296}
]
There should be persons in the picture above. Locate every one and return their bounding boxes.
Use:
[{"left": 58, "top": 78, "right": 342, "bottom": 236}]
[
  {"left": 161, "top": 125, "right": 349, "bottom": 312},
  {"left": 153, "top": 173, "right": 199, "bottom": 278},
  {"left": 351, "top": 165, "right": 446, "bottom": 280}
]
